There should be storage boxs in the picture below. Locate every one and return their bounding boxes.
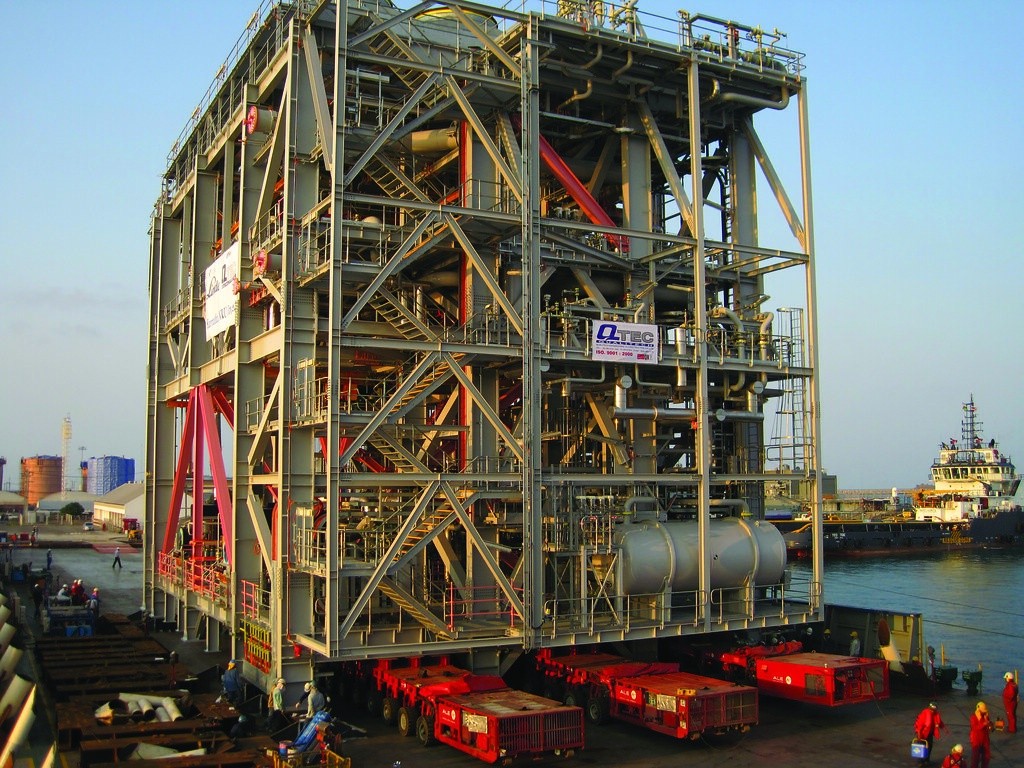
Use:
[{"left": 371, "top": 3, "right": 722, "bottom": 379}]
[{"left": 911, "top": 738, "right": 928, "bottom": 758}]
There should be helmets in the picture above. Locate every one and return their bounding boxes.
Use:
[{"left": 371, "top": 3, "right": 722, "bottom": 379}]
[
  {"left": 929, "top": 701, "right": 938, "bottom": 709},
  {"left": 34, "top": 584, "right": 39, "bottom": 588},
  {"left": 824, "top": 629, "right": 832, "bottom": 634},
  {"left": 952, "top": 744, "right": 963, "bottom": 753},
  {"left": 274, "top": 678, "right": 286, "bottom": 684},
  {"left": 63, "top": 579, "right": 99, "bottom": 592},
  {"left": 1003, "top": 672, "right": 1015, "bottom": 679},
  {"left": 116, "top": 547, "right": 120, "bottom": 549},
  {"left": 48, "top": 549, "right": 51, "bottom": 551},
  {"left": 977, "top": 702, "right": 988, "bottom": 712},
  {"left": 850, "top": 632, "right": 859, "bottom": 637},
  {"left": 304, "top": 681, "right": 317, "bottom": 693}
]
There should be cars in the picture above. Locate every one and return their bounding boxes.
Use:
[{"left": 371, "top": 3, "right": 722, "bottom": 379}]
[{"left": 83, "top": 522, "right": 94, "bottom": 531}]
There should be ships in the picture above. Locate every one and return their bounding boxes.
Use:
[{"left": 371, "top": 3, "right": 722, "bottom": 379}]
[{"left": 766, "top": 393, "right": 1024, "bottom": 562}]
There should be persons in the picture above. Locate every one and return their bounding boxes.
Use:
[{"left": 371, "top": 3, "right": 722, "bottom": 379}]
[
  {"left": 306, "top": 680, "right": 326, "bottom": 719},
  {"left": 46, "top": 548, "right": 52, "bottom": 571},
  {"left": 941, "top": 743, "right": 968, "bottom": 768},
  {"left": 223, "top": 659, "right": 245, "bottom": 713},
  {"left": 270, "top": 678, "right": 289, "bottom": 740},
  {"left": 821, "top": 628, "right": 834, "bottom": 653},
  {"left": 112, "top": 546, "right": 124, "bottom": 568},
  {"left": 228, "top": 714, "right": 253, "bottom": 738},
  {"left": 295, "top": 682, "right": 331, "bottom": 710},
  {"left": 11, "top": 526, "right": 39, "bottom": 546},
  {"left": 848, "top": 630, "right": 860, "bottom": 657},
  {"left": 969, "top": 701, "right": 994, "bottom": 768},
  {"left": 1002, "top": 671, "right": 1019, "bottom": 734},
  {"left": 31, "top": 579, "right": 101, "bottom": 621},
  {"left": 914, "top": 701, "right": 949, "bottom": 768}
]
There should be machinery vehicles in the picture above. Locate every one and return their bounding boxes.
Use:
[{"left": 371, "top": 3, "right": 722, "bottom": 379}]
[
  {"left": 539, "top": 644, "right": 758, "bottom": 746},
  {"left": 129, "top": 527, "right": 143, "bottom": 548},
  {"left": 373, "top": 654, "right": 583, "bottom": 766},
  {"left": 683, "top": 625, "right": 893, "bottom": 708}
]
